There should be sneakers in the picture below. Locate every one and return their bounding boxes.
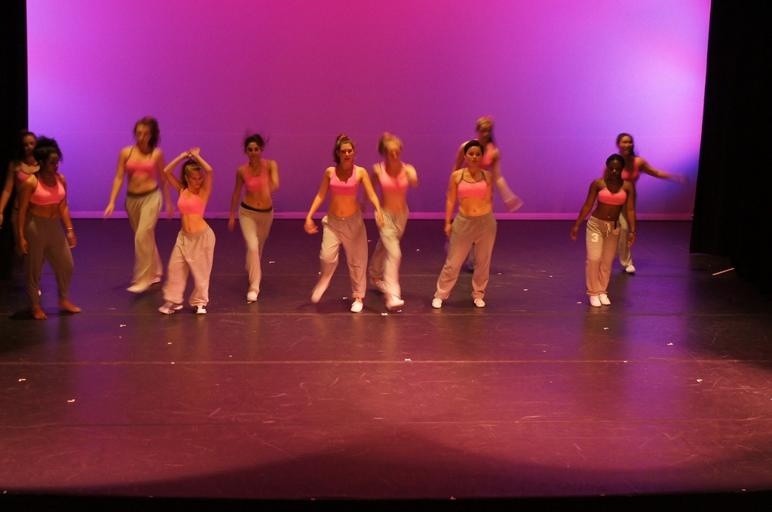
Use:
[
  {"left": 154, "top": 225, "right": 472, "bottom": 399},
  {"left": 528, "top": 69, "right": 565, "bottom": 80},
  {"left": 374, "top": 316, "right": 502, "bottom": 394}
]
[
  {"left": 127, "top": 283, "right": 150, "bottom": 294},
  {"left": 474, "top": 298, "right": 486, "bottom": 306},
  {"left": 589, "top": 294, "right": 609, "bottom": 307},
  {"left": 351, "top": 277, "right": 405, "bottom": 312},
  {"left": 194, "top": 306, "right": 208, "bottom": 315},
  {"left": 432, "top": 297, "right": 444, "bottom": 308},
  {"left": 246, "top": 290, "right": 257, "bottom": 302},
  {"left": 312, "top": 287, "right": 323, "bottom": 304},
  {"left": 625, "top": 264, "right": 635, "bottom": 272},
  {"left": 159, "top": 302, "right": 183, "bottom": 314}
]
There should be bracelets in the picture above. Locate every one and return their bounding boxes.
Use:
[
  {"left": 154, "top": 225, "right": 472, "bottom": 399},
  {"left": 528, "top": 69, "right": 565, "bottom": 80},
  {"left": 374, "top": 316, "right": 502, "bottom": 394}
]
[
  {"left": 66, "top": 228, "right": 76, "bottom": 231},
  {"left": 628, "top": 231, "right": 635, "bottom": 235}
]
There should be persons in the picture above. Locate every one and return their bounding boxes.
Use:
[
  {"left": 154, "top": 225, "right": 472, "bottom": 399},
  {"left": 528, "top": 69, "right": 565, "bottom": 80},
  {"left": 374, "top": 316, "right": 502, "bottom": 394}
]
[
  {"left": 0, "top": 131, "right": 42, "bottom": 296},
  {"left": 226, "top": 132, "right": 279, "bottom": 303},
  {"left": 612, "top": 132, "right": 680, "bottom": 273},
  {"left": 361, "top": 132, "right": 419, "bottom": 308},
  {"left": 430, "top": 138, "right": 498, "bottom": 308},
  {"left": 105, "top": 115, "right": 175, "bottom": 295},
  {"left": 16, "top": 136, "right": 84, "bottom": 320},
  {"left": 568, "top": 152, "right": 637, "bottom": 308},
  {"left": 453, "top": 115, "right": 523, "bottom": 275},
  {"left": 300, "top": 133, "right": 386, "bottom": 313},
  {"left": 155, "top": 146, "right": 216, "bottom": 316}
]
